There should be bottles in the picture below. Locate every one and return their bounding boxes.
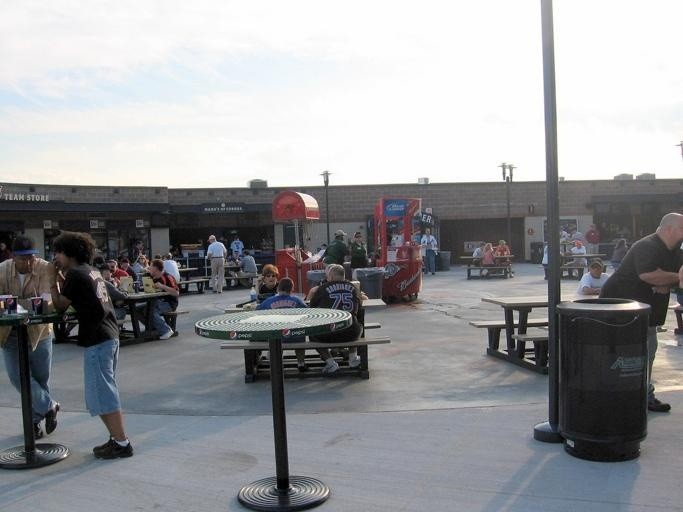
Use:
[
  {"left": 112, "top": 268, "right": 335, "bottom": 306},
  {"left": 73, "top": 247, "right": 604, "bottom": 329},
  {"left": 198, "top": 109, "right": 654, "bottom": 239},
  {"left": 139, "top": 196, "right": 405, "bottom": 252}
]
[
  {"left": 250, "top": 286, "right": 257, "bottom": 309},
  {"left": 503, "top": 249, "right": 506, "bottom": 256},
  {"left": 235, "top": 258, "right": 238, "bottom": 266},
  {"left": 497, "top": 250, "right": 500, "bottom": 256}
]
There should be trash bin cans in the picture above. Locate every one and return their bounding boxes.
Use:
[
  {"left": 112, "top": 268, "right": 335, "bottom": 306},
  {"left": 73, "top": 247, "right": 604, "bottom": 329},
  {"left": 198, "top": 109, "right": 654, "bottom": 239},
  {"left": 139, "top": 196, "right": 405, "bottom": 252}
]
[
  {"left": 438, "top": 251, "right": 451, "bottom": 271},
  {"left": 530, "top": 242, "right": 544, "bottom": 264},
  {"left": 355, "top": 268, "right": 383, "bottom": 300},
  {"left": 306, "top": 269, "right": 327, "bottom": 289},
  {"left": 554, "top": 297, "right": 651, "bottom": 463},
  {"left": 342, "top": 261, "right": 352, "bottom": 281}
]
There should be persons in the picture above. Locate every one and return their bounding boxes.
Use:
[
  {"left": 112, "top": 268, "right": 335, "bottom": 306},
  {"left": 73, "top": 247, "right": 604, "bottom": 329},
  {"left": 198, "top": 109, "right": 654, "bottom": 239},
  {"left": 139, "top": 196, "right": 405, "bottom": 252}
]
[
  {"left": 92, "top": 240, "right": 182, "bottom": 345},
  {"left": 203, "top": 231, "right": 246, "bottom": 293},
  {"left": 597, "top": 212, "right": 683, "bottom": 412},
  {"left": 319, "top": 229, "right": 351, "bottom": 268},
  {"left": 574, "top": 257, "right": 611, "bottom": 296},
  {"left": 303, "top": 243, "right": 328, "bottom": 265},
  {"left": 472, "top": 239, "right": 511, "bottom": 273},
  {"left": 45, "top": 231, "right": 134, "bottom": 459},
  {"left": 422, "top": 227, "right": 439, "bottom": 276},
  {"left": 537, "top": 219, "right": 632, "bottom": 281},
  {"left": 0, "top": 235, "right": 62, "bottom": 440},
  {"left": 237, "top": 251, "right": 258, "bottom": 287},
  {"left": 349, "top": 231, "right": 369, "bottom": 272},
  {"left": 249, "top": 262, "right": 370, "bottom": 373}
]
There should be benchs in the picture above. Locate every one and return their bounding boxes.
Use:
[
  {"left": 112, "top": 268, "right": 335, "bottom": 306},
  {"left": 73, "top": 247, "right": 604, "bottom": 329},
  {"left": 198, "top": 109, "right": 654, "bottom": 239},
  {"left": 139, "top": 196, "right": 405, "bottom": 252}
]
[
  {"left": 221, "top": 336, "right": 391, "bottom": 384},
  {"left": 261, "top": 322, "right": 381, "bottom": 360},
  {"left": 56, "top": 265, "right": 258, "bottom": 343},
  {"left": 460, "top": 253, "right": 683, "bottom": 374}
]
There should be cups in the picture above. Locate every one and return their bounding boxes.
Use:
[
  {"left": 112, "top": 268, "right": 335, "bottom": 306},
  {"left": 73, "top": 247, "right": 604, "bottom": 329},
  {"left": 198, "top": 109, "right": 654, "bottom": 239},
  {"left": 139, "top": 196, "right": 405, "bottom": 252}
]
[
  {"left": 404, "top": 241, "right": 409, "bottom": 246},
  {"left": 31, "top": 297, "right": 43, "bottom": 315},
  {"left": 5, "top": 296, "right": 18, "bottom": 314},
  {"left": 133, "top": 282, "right": 140, "bottom": 293}
]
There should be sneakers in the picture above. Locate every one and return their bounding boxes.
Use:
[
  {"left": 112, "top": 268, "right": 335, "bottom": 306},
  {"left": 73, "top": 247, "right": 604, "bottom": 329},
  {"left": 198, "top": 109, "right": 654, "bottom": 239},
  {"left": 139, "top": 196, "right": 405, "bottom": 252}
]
[
  {"left": 298, "top": 350, "right": 361, "bottom": 373},
  {"left": 160, "top": 330, "right": 174, "bottom": 340},
  {"left": 649, "top": 399, "right": 670, "bottom": 411},
  {"left": 93, "top": 436, "right": 133, "bottom": 459}
]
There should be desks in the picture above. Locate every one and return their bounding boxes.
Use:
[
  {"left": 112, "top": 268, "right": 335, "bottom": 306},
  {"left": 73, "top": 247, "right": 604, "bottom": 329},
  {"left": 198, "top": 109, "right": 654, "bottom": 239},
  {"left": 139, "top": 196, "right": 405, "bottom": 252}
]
[
  {"left": 195, "top": 308, "right": 352, "bottom": 512},
  {"left": 0, "top": 308, "right": 79, "bottom": 469}
]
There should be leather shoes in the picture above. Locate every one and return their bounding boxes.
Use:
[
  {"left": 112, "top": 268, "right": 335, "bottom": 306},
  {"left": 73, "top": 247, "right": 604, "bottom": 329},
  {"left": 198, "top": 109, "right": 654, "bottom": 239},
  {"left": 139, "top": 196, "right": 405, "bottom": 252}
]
[
  {"left": 46, "top": 400, "right": 60, "bottom": 434},
  {"left": 34, "top": 423, "right": 42, "bottom": 439}
]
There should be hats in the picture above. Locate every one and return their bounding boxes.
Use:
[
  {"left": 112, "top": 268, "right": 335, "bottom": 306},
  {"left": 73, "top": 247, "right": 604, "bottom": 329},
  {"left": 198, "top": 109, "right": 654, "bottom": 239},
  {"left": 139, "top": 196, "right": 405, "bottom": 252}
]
[
  {"left": 335, "top": 230, "right": 348, "bottom": 237},
  {"left": 207, "top": 235, "right": 216, "bottom": 242},
  {"left": 13, "top": 237, "right": 39, "bottom": 255},
  {"left": 589, "top": 258, "right": 603, "bottom": 268}
]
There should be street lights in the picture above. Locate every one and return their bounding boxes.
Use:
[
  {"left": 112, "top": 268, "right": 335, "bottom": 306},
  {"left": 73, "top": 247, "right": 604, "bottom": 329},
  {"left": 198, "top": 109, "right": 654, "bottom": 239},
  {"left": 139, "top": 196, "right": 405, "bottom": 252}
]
[
  {"left": 319, "top": 170, "right": 331, "bottom": 246},
  {"left": 499, "top": 162, "right": 517, "bottom": 243}
]
